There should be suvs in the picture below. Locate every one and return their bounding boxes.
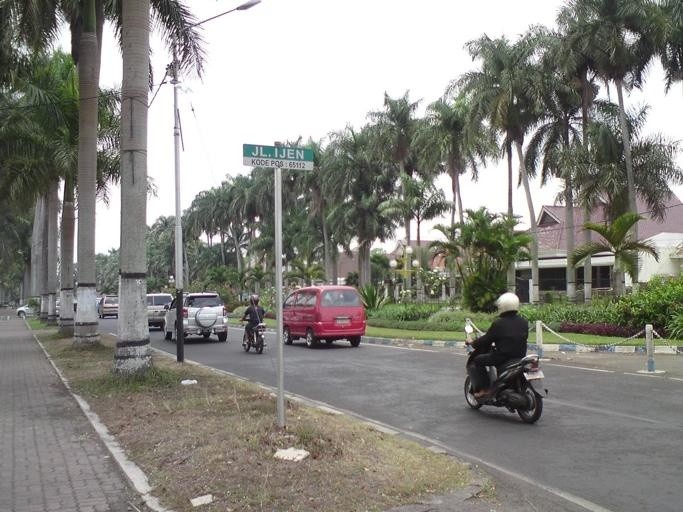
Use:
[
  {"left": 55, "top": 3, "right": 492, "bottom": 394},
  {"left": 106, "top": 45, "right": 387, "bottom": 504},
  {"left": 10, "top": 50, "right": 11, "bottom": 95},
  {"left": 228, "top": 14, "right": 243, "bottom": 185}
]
[{"left": 165, "top": 291, "right": 228, "bottom": 344}]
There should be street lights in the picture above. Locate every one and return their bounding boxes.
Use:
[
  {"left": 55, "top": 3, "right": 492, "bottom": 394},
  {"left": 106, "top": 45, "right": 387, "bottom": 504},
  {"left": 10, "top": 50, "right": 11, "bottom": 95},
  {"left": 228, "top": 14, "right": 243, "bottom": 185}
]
[{"left": 172, "top": 0, "right": 261, "bottom": 362}]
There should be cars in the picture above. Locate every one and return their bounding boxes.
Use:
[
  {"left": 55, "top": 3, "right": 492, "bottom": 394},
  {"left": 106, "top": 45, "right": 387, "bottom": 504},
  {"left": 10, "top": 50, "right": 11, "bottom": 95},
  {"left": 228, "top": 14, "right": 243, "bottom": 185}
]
[
  {"left": 16, "top": 295, "right": 118, "bottom": 319},
  {"left": 146, "top": 293, "right": 171, "bottom": 331}
]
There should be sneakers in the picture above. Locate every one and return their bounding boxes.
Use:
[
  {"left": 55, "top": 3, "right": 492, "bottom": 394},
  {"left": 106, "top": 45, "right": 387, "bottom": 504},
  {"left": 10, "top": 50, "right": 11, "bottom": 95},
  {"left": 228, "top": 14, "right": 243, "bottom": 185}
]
[{"left": 472, "top": 390, "right": 491, "bottom": 399}]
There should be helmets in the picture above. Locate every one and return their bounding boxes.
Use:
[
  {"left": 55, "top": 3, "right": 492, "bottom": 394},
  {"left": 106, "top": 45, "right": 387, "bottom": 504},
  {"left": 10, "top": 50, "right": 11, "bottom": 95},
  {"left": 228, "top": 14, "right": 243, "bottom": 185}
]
[
  {"left": 248, "top": 294, "right": 261, "bottom": 306},
  {"left": 496, "top": 291, "right": 521, "bottom": 316}
]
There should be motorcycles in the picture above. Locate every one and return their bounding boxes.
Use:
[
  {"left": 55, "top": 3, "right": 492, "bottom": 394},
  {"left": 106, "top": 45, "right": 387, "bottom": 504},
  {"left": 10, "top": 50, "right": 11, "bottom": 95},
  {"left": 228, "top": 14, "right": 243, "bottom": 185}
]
[
  {"left": 239, "top": 318, "right": 267, "bottom": 354},
  {"left": 465, "top": 325, "right": 548, "bottom": 424}
]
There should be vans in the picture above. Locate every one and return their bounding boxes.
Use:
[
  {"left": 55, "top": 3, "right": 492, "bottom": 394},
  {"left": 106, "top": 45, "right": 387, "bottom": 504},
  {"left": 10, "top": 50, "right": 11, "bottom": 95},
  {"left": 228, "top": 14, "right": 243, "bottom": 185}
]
[{"left": 283, "top": 285, "right": 366, "bottom": 348}]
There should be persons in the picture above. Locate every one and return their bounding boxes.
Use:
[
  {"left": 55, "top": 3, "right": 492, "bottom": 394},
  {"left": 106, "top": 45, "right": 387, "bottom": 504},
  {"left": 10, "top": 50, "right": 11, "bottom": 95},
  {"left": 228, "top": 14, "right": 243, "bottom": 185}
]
[
  {"left": 463, "top": 290, "right": 529, "bottom": 399},
  {"left": 237, "top": 292, "right": 265, "bottom": 346}
]
